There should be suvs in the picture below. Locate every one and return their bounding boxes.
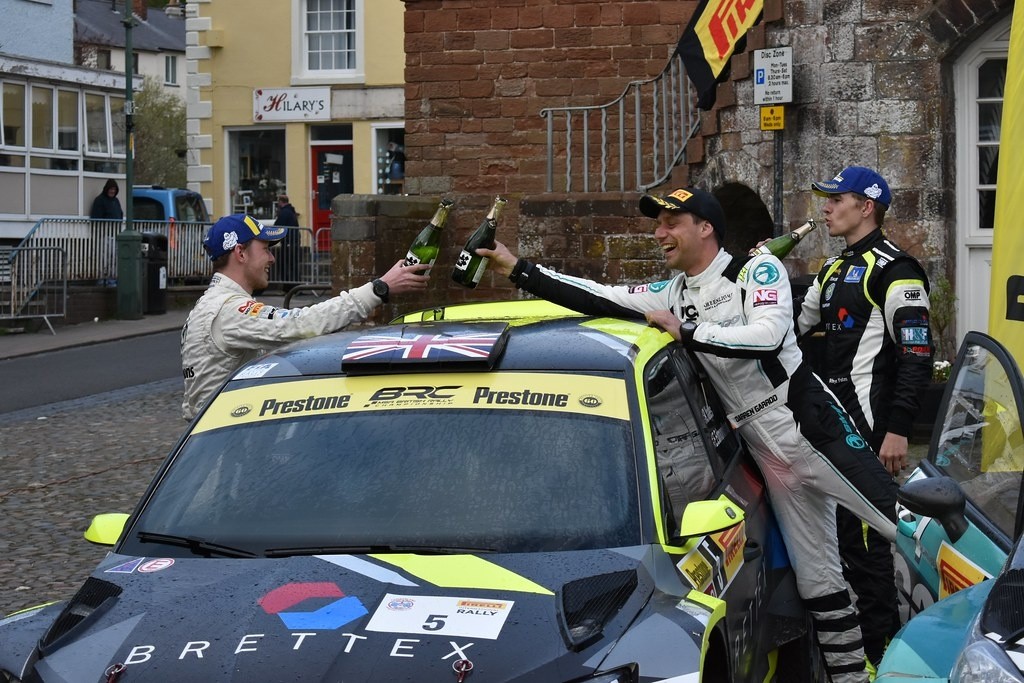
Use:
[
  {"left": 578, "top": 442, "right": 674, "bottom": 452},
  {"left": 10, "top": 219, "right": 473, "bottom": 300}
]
[{"left": 132, "top": 184, "right": 211, "bottom": 286}]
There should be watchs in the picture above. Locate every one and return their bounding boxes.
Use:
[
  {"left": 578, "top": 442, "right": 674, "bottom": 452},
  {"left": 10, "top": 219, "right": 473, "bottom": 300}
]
[
  {"left": 372, "top": 278, "right": 392, "bottom": 307},
  {"left": 680, "top": 319, "right": 698, "bottom": 349}
]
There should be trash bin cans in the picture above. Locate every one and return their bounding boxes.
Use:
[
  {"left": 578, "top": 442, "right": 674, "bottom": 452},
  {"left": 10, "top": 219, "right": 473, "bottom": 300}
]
[{"left": 142, "top": 231, "right": 168, "bottom": 315}]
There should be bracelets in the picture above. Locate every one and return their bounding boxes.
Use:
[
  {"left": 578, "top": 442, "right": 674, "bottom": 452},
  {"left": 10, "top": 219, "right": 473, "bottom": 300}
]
[{"left": 514, "top": 259, "right": 535, "bottom": 289}]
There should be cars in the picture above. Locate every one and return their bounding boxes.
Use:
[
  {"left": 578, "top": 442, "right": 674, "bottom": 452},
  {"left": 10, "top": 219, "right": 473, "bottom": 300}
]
[
  {"left": 1, "top": 300, "right": 831, "bottom": 683},
  {"left": 872, "top": 331, "right": 1024, "bottom": 682}
]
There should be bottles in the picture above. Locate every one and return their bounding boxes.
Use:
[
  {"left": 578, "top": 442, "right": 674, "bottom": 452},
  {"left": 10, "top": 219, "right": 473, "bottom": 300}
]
[
  {"left": 751, "top": 218, "right": 817, "bottom": 259},
  {"left": 451, "top": 196, "right": 507, "bottom": 289},
  {"left": 403, "top": 199, "right": 454, "bottom": 275}
]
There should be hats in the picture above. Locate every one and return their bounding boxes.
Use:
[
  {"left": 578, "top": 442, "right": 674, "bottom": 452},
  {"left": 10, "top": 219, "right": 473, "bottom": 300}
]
[
  {"left": 639, "top": 186, "right": 724, "bottom": 237},
  {"left": 811, "top": 168, "right": 891, "bottom": 207},
  {"left": 202, "top": 213, "right": 289, "bottom": 262}
]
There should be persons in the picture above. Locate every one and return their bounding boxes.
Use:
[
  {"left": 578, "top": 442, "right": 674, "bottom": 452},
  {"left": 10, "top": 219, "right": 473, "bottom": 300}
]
[
  {"left": 182, "top": 213, "right": 431, "bottom": 429},
  {"left": 253, "top": 179, "right": 276, "bottom": 219},
  {"left": 749, "top": 166, "right": 939, "bottom": 683},
  {"left": 268, "top": 193, "right": 304, "bottom": 298},
  {"left": 474, "top": 181, "right": 902, "bottom": 683},
  {"left": 89, "top": 177, "right": 123, "bottom": 288}
]
